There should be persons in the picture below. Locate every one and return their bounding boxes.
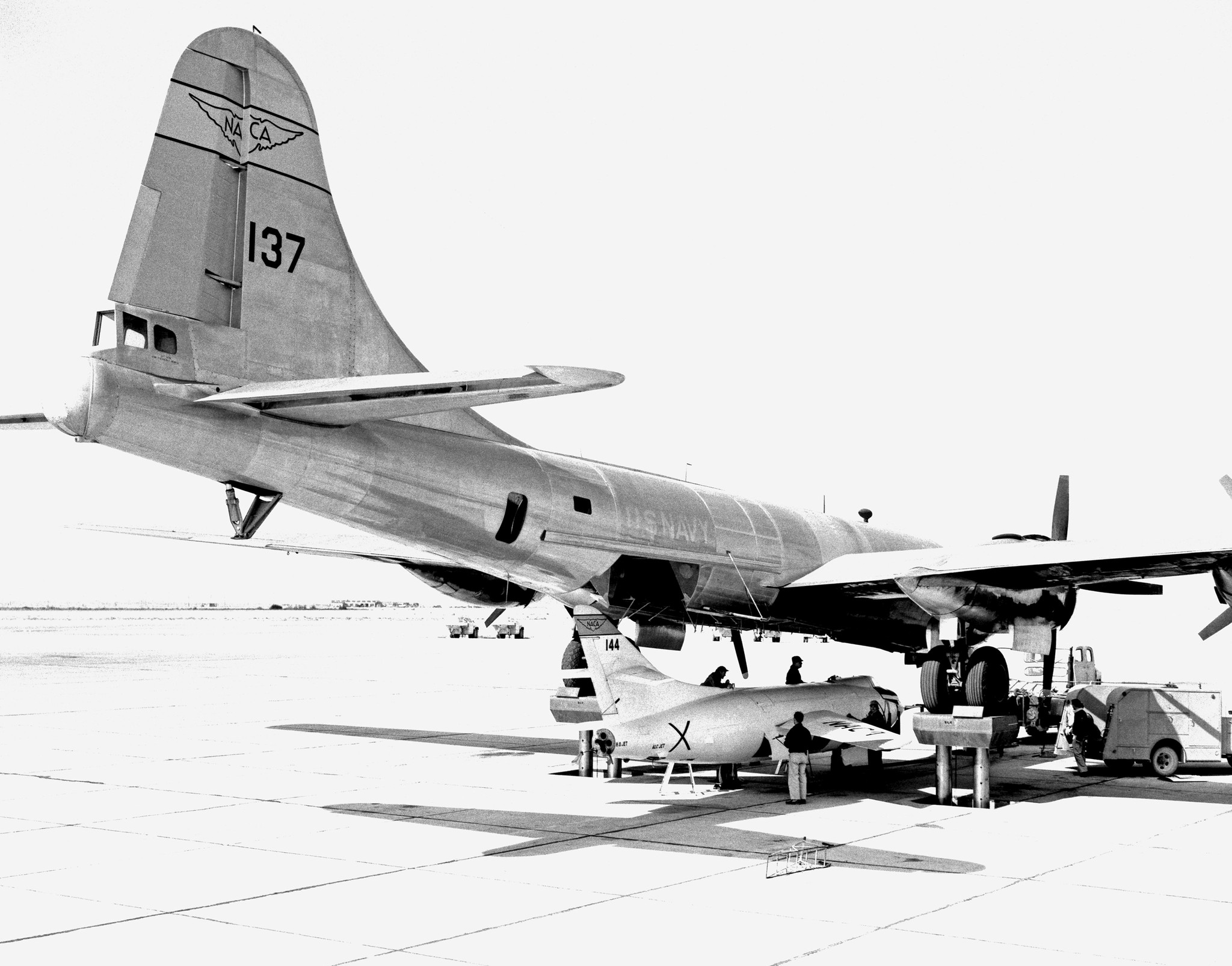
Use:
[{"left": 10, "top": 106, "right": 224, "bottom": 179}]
[
  {"left": 1063, "top": 698, "right": 1090, "bottom": 777},
  {"left": 785, "top": 656, "right": 808, "bottom": 685},
  {"left": 784, "top": 711, "right": 812, "bottom": 805},
  {"left": 700, "top": 666, "right": 730, "bottom": 688}
]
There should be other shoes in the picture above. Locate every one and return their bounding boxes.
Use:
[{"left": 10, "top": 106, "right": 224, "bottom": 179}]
[
  {"left": 1073, "top": 770, "right": 1088, "bottom": 777},
  {"left": 785, "top": 799, "right": 806, "bottom": 805}
]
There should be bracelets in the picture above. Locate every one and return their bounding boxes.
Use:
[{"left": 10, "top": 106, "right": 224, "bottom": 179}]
[{"left": 1066, "top": 725, "right": 1071, "bottom": 729}]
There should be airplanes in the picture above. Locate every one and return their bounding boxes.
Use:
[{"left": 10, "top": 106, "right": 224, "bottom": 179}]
[
  {"left": 0, "top": 25, "right": 1232, "bottom": 707},
  {"left": 570, "top": 607, "right": 906, "bottom": 785}
]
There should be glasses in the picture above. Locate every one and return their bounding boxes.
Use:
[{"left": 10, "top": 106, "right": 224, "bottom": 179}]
[{"left": 870, "top": 705, "right": 877, "bottom": 708}]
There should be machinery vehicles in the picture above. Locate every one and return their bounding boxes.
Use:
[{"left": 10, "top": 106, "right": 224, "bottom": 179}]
[
  {"left": 1054, "top": 682, "right": 1232, "bottom": 775},
  {"left": 1007, "top": 647, "right": 1102, "bottom": 736}
]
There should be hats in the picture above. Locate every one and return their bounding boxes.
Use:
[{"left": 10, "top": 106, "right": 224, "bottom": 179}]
[
  {"left": 869, "top": 701, "right": 878, "bottom": 706},
  {"left": 717, "top": 666, "right": 729, "bottom": 672},
  {"left": 792, "top": 656, "right": 804, "bottom": 663}
]
